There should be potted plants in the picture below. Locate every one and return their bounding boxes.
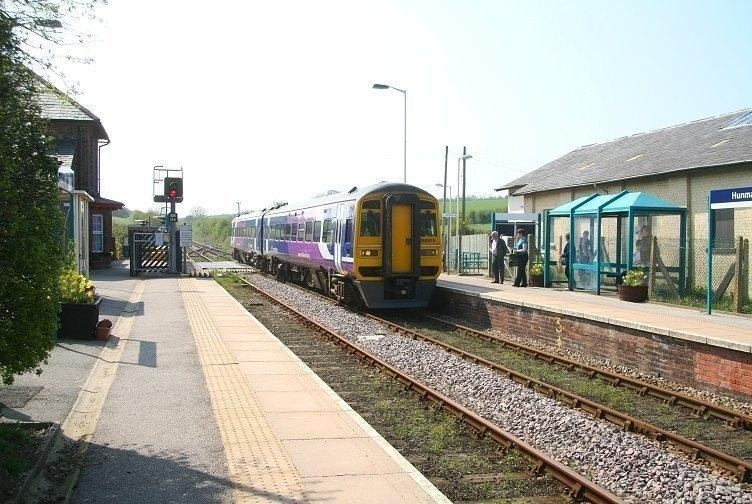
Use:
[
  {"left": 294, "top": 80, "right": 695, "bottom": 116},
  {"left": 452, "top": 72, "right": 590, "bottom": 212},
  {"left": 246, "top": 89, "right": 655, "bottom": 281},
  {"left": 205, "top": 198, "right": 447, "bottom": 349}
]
[
  {"left": 617, "top": 265, "right": 649, "bottom": 303},
  {"left": 53, "top": 270, "right": 102, "bottom": 343},
  {"left": 530, "top": 261, "right": 554, "bottom": 289}
]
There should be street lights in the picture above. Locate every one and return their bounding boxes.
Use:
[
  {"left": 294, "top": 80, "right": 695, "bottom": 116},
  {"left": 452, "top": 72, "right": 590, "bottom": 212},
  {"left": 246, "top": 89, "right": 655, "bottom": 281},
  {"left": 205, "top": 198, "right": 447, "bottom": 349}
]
[
  {"left": 456, "top": 155, "right": 472, "bottom": 236},
  {"left": 436, "top": 184, "right": 451, "bottom": 236},
  {"left": 372, "top": 84, "right": 406, "bottom": 183}
]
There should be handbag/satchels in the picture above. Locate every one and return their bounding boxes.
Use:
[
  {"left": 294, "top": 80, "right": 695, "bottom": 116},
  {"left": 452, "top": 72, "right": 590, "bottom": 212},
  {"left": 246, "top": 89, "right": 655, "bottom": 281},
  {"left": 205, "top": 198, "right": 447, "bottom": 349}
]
[{"left": 508, "top": 247, "right": 519, "bottom": 266}]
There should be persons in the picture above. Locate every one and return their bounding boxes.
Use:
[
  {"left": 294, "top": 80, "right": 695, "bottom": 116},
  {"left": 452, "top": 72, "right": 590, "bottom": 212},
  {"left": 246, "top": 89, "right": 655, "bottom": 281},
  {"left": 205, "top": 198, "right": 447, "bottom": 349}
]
[
  {"left": 557, "top": 232, "right": 577, "bottom": 290},
  {"left": 511, "top": 228, "right": 528, "bottom": 287},
  {"left": 575, "top": 230, "right": 591, "bottom": 291},
  {"left": 488, "top": 230, "right": 507, "bottom": 284},
  {"left": 632, "top": 224, "right": 660, "bottom": 292}
]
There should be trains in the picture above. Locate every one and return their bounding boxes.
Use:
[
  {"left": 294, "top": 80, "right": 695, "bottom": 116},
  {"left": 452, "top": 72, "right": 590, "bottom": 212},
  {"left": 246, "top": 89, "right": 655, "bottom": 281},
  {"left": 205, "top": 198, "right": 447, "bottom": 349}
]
[{"left": 230, "top": 181, "right": 442, "bottom": 310}]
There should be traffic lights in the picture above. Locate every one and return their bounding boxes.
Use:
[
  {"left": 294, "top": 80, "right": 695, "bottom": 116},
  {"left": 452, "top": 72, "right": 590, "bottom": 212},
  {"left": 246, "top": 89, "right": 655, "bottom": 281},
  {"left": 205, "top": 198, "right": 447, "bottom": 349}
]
[{"left": 164, "top": 177, "right": 183, "bottom": 203}]
